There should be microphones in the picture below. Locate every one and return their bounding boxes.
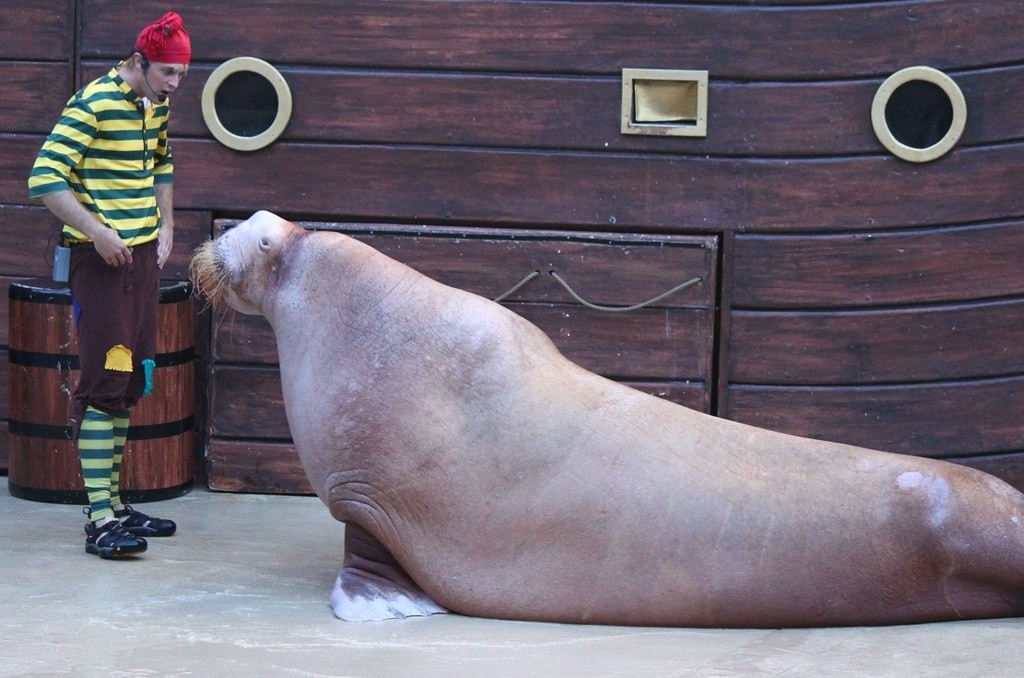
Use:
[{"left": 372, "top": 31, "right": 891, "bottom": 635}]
[{"left": 143, "top": 69, "right": 166, "bottom": 102}]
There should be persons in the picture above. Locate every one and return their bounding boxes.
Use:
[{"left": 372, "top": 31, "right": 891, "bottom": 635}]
[{"left": 28, "top": 12, "right": 190, "bottom": 558}]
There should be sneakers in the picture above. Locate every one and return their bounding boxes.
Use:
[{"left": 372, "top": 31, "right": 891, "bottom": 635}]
[
  {"left": 82, "top": 504, "right": 176, "bottom": 537},
  {"left": 85, "top": 520, "right": 147, "bottom": 559}
]
